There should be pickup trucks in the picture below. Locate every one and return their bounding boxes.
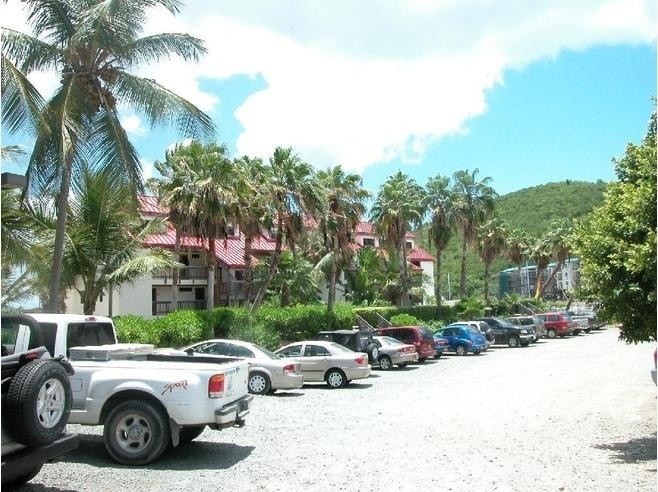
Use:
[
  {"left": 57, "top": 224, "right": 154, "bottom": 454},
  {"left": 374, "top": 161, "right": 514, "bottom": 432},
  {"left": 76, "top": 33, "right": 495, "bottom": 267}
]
[{"left": 1, "top": 313, "right": 253, "bottom": 465}]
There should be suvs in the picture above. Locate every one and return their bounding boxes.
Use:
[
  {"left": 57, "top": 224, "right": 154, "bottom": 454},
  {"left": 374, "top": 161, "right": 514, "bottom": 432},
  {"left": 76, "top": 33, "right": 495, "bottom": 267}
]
[{"left": 1, "top": 312, "right": 79, "bottom": 485}]
